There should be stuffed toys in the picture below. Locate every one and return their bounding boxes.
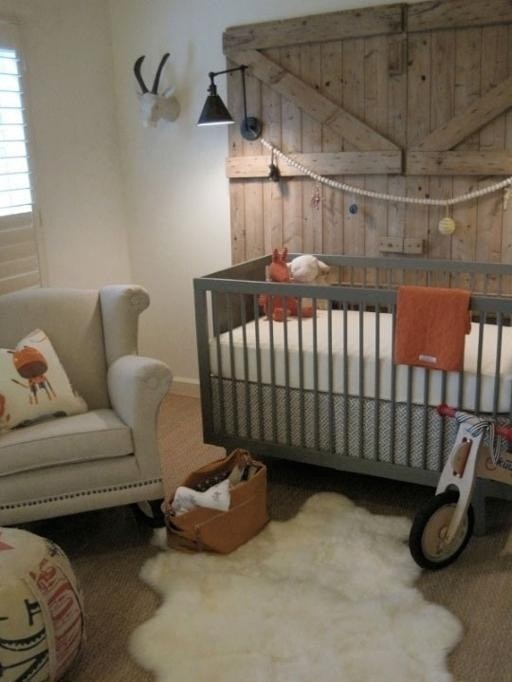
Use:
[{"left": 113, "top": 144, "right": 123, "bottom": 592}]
[
  {"left": 255, "top": 245, "right": 313, "bottom": 322},
  {"left": 284, "top": 254, "right": 331, "bottom": 310}
]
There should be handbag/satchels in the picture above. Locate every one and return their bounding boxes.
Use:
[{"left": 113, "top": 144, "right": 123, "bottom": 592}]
[{"left": 161, "top": 449, "right": 269, "bottom": 553}]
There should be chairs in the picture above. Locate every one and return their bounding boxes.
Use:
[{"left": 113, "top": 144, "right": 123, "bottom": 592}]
[{"left": 0, "top": 285, "right": 172, "bottom": 529}]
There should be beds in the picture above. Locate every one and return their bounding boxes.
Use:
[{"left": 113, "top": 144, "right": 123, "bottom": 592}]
[{"left": 194, "top": 253, "right": 512, "bottom": 536}]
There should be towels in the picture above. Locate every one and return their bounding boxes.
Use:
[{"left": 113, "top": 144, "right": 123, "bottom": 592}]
[{"left": 393, "top": 286, "right": 471, "bottom": 370}]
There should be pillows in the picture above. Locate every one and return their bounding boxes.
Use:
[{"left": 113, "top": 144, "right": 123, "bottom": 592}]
[{"left": 0, "top": 328, "right": 89, "bottom": 436}]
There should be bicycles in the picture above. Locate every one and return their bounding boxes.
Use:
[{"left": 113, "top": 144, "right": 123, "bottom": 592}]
[{"left": 406, "top": 402, "right": 511, "bottom": 570}]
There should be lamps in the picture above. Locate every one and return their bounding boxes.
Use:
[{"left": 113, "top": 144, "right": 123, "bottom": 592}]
[{"left": 198, "top": 65, "right": 262, "bottom": 140}]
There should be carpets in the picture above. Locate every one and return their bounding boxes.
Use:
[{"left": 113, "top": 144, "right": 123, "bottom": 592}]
[{"left": 127, "top": 491, "right": 466, "bottom": 682}]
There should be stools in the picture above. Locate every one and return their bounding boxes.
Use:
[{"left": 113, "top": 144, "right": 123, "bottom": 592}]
[{"left": 0, "top": 527, "right": 86, "bottom": 682}]
